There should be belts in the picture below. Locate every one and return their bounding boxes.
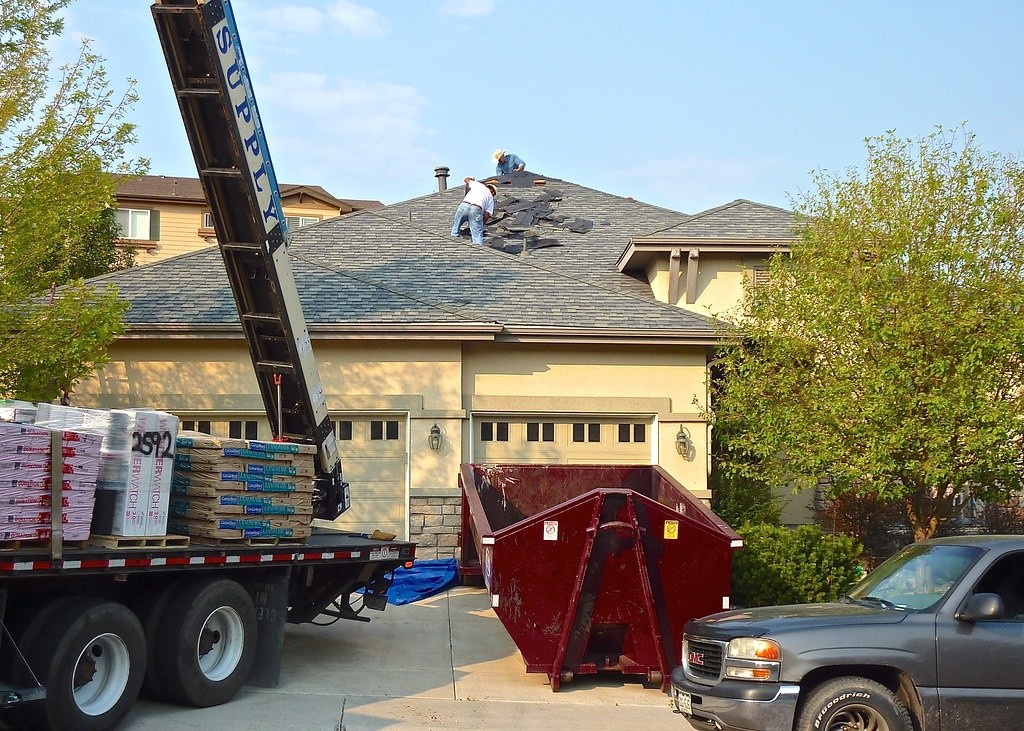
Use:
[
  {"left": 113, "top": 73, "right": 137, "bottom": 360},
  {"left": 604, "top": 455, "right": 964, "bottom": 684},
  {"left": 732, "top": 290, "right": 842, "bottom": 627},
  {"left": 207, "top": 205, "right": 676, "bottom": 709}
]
[{"left": 461, "top": 201, "right": 481, "bottom": 209}]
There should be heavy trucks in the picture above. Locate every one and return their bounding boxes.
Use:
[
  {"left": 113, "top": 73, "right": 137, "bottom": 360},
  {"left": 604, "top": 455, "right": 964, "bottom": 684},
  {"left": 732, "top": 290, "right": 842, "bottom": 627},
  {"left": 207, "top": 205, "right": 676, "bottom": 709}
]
[{"left": 0, "top": 0, "right": 420, "bottom": 731}]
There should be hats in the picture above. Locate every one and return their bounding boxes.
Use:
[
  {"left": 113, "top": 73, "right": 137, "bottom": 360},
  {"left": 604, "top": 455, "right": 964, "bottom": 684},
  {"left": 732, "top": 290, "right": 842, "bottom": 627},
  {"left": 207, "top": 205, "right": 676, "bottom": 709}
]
[
  {"left": 487, "top": 184, "right": 497, "bottom": 196},
  {"left": 493, "top": 150, "right": 506, "bottom": 159}
]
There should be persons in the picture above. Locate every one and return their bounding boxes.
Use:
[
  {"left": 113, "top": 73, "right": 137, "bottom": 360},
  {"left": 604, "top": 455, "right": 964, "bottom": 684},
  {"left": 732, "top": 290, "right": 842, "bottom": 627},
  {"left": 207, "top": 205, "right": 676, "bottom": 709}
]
[
  {"left": 450, "top": 176, "right": 497, "bottom": 247},
  {"left": 492, "top": 147, "right": 526, "bottom": 176}
]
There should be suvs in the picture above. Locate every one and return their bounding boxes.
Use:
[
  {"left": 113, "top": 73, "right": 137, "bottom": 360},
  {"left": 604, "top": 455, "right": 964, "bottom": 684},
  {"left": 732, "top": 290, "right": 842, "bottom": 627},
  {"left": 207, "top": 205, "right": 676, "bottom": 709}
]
[{"left": 671, "top": 535, "right": 1024, "bottom": 731}]
